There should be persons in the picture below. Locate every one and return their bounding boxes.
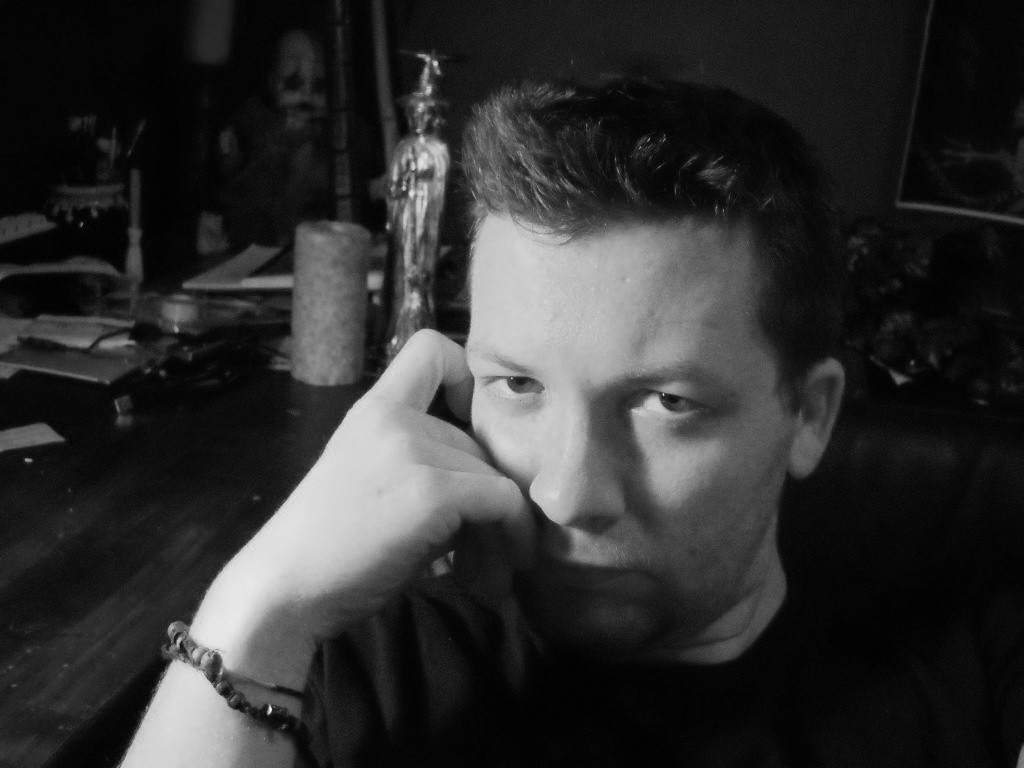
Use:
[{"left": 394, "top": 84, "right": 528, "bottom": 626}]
[
  {"left": 117, "top": 79, "right": 1024, "bottom": 768},
  {"left": 383, "top": 51, "right": 452, "bottom": 364},
  {"left": 862, "top": 291, "right": 980, "bottom": 408}
]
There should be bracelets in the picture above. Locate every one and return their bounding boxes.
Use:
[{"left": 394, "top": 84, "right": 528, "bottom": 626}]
[{"left": 160, "top": 620, "right": 314, "bottom": 748}]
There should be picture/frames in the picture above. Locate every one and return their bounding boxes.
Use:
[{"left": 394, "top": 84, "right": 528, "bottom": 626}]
[{"left": 888, "top": 0, "right": 1024, "bottom": 240}]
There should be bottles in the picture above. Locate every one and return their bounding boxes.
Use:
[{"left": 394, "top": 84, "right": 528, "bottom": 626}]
[{"left": 43, "top": 186, "right": 128, "bottom": 269}]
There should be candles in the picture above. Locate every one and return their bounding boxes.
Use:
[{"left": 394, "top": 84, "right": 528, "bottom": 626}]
[{"left": 293, "top": 219, "right": 372, "bottom": 385}]
